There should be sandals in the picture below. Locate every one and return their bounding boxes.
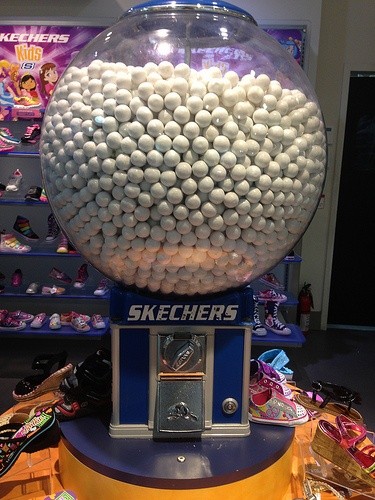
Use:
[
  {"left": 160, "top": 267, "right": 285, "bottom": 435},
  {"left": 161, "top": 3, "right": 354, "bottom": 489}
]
[{"left": 294, "top": 382, "right": 363, "bottom": 424}]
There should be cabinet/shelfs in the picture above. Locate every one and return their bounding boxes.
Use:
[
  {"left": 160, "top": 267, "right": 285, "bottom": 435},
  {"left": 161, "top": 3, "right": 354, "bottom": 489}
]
[{"left": 0, "top": 150, "right": 306, "bottom": 348}]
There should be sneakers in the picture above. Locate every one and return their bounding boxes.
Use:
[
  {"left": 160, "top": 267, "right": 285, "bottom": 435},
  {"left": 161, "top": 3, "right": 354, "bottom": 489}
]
[
  {"left": 250, "top": 388, "right": 307, "bottom": 426},
  {"left": 250, "top": 358, "right": 286, "bottom": 384},
  {"left": 247, "top": 250, "right": 300, "bottom": 335},
  {"left": 251, "top": 372, "right": 292, "bottom": 399},
  {"left": 0, "top": 123, "right": 111, "bottom": 331}
]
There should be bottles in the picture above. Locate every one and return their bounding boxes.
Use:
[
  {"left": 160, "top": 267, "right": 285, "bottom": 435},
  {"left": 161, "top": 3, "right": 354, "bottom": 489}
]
[{"left": 39, "top": 0, "right": 327, "bottom": 298}]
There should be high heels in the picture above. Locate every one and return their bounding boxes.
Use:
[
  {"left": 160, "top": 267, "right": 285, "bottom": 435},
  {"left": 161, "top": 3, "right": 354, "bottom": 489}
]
[{"left": 311, "top": 413, "right": 375, "bottom": 487}]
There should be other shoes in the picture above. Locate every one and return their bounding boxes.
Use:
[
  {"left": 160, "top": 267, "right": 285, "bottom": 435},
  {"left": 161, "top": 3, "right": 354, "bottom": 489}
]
[{"left": 0, "top": 347, "right": 110, "bottom": 477}]
